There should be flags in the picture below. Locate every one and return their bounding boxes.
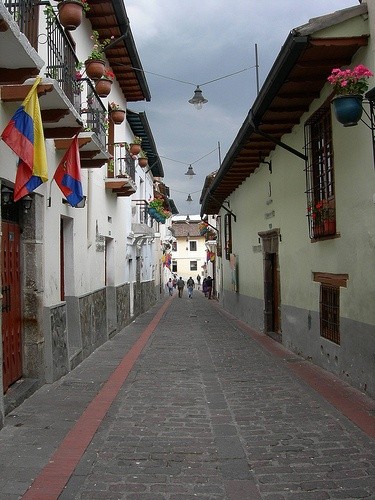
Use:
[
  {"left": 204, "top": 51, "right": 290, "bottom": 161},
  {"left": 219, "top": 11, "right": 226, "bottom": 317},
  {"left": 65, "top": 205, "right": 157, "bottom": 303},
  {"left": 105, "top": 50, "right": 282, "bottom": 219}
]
[
  {"left": 164, "top": 255, "right": 172, "bottom": 271},
  {"left": 3, "top": 77, "right": 49, "bottom": 202},
  {"left": 53, "top": 136, "right": 83, "bottom": 207},
  {"left": 207, "top": 252, "right": 214, "bottom": 261}
]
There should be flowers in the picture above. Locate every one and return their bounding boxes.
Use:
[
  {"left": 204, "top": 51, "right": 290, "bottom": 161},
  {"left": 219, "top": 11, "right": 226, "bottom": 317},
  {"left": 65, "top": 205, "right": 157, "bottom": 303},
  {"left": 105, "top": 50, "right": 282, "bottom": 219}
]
[
  {"left": 133, "top": 136, "right": 142, "bottom": 144},
  {"left": 64, "top": 0, "right": 90, "bottom": 16},
  {"left": 308, "top": 201, "right": 335, "bottom": 223},
  {"left": 327, "top": 64, "right": 374, "bottom": 95},
  {"left": 88, "top": 31, "right": 114, "bottom": 60},
  {"left": 148, "top": 197, "right": 172, "bottom": 219},
  {"left": 111, "top": 102, "right": 121, "bottom": 110},
  {"left": 101, "top": 66, "right": 114, "bottom": 81}
]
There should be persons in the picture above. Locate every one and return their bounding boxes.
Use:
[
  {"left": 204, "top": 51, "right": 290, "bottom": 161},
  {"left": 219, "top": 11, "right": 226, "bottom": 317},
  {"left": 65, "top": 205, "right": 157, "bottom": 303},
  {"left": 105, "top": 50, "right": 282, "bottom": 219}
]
[
  {"left": 206, "top": 276, "right": 215, "bottom": 300},
  {"left": 204, "top": 281, "right": 209, "bottom": 297},
  {"left": 172, "top": 276, "right": 177, "bottom": 289},
  {"left": 203, "top": 278, "right": 207, "bottom": 292},
  {"left": 187, "top": 277, "right": 195, "bottom": 299},
  {"left": 177, "top": 277, "right": 185, "bottom": 298},
  {"left": 167, "top": 278, "right": 174, "bottom": 297},
  {"left": 196, "top": 274, "right": 201, "bottom": 285}
]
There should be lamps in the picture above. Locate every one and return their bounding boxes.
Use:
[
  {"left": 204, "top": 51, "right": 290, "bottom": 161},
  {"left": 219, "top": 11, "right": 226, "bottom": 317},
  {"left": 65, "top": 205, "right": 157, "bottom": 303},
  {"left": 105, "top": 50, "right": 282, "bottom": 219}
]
[
  {"left": 187, "top": 194, "right": 193, "bottom": 202},
  {"left": 22, "top": 195, "right": 33, "bottom": 210},
  {"left": 185, "top": 164, "right": 196, "bottom": 179},
  {"left": 1, "top": 187, "right": 13, "bottom": 205},
  {"left": 186, "top": 215, "right": 190, "bottom": 220},
  {"left": 188, "top": 85, "right": 208, "bottom": 109}
]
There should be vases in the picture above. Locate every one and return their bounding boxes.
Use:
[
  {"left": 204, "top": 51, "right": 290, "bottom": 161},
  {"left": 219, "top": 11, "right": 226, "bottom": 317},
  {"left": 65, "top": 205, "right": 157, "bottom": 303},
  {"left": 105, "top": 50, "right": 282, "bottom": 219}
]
[
  {"left": 95, "top": 79, "right": 113, "bottom": 97},
  {"left": 84, "top": 60, "right": 106, "bottom": 80},
  {"left": 330, "top": 95, "right": 363, "bottom": 127},
  {"left": 313, "top": 221, "right": 335, "bottom": 235},
  {"left": 57, "top": 1, "right": 84, "bottom": 30},
  {"left": 111, "top": 110, "right": 125, "bottom": 124},
  {"left": 130, "top": 144, "right": 141, "bottom": 155},
  {"left": 148, "top": 208, "right": 166, "bottom": 224}
]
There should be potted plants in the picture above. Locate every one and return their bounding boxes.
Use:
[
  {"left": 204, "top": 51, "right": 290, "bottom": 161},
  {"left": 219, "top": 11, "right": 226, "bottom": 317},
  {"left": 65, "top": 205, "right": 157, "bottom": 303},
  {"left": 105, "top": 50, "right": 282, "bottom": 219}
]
[{"left": 138, "top": 152, "right": 148, "bottom": 167}]
